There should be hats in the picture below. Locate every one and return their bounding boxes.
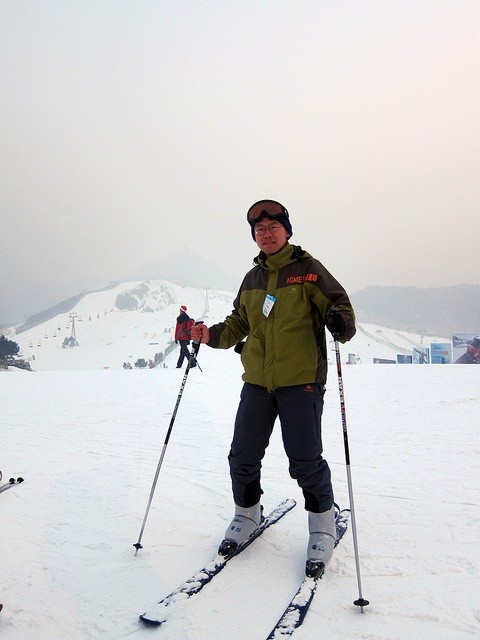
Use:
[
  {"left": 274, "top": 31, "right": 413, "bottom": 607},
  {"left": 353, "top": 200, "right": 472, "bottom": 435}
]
[
  {"left": 180, "top": 306, "right": 186, "bottom": 313},
  {"left": 251, "top": 212, "right": 292, "bottom": 242}
]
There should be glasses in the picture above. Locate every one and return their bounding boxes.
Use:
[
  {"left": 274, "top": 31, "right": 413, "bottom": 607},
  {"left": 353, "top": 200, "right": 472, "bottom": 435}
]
[{"left": 247, "top": 200, "right": 289, "bottom": 226}]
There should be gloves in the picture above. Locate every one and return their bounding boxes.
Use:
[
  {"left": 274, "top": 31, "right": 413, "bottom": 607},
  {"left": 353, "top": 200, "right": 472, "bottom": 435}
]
[
  {"left": 175, "top": 339, "right": 178, "bottom": 344},
  {"left": 324, "top": 313, "right": 347, "bottom": 337}
]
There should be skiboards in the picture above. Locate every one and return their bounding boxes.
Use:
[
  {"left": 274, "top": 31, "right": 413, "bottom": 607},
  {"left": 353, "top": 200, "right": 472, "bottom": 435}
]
[{"left": 139, "top": 499, "right": 351, "bottom": 640}]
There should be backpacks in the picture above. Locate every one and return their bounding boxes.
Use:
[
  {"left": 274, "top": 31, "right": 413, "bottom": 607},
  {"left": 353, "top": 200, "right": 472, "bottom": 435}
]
[{"left": 177, "top": 318, "right": 195, "bottom": 341}]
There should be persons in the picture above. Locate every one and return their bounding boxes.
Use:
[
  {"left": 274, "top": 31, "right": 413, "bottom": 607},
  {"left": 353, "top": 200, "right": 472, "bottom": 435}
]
[
  {"left": 175, "top": 305, "right": 197, "bottom": 368},
  {"left": 190, "top": 200, "right": 356, "bottom": 565}
]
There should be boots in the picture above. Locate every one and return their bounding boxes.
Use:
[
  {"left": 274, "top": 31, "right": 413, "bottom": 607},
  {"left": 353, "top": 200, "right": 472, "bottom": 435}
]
[
  {"left": 307, "top": 503, "right": 335, "bottom": 565},
  {"left": 225, "top": 500, "right": 261, "bottom": 546}
]
